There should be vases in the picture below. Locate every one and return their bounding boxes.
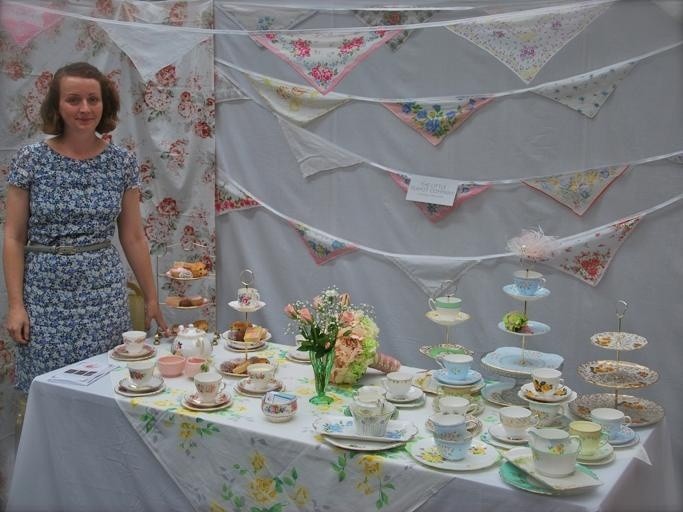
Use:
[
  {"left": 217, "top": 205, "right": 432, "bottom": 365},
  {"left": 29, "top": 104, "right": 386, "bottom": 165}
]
[{"left": 308, "top": 349, "right": 335, "bottom": 406}]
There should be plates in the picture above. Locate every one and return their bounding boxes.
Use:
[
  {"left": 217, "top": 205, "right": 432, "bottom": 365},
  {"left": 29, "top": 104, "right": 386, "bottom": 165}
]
[
  {"left": 220, "top": 325, "right": 272, "bottom": 349},
  {"left": 480, "top": 285, "right": 564, "bottom": 374},
  {"left": 424, "top": 311, "right": 470, "bottom": 327},
  {"left": 567, "top": 329, "right": 665, "bottom": 426},
  {"left": 164, "top": 269, "right": 204, "bottom": 281},
  {"left": 317, "top": 417, "right": 418, "bottom": 443},
  {"left": 311, "top": 418, "right": 418, "bottom": 452},
  {"left": 500, "top": 424, "right": 640, "bottom": 498},
  {"left": 227, "top": 300, "right": 266, "bottom": 314},
  {"left": 418, "top": 344, "right": 474, "bottom": 361},
  {"left": 107, "top": 341, "right": 311, "bottom": 411},
  {"left": 175, "top": 301, "right": 209, "bottom": 310}
]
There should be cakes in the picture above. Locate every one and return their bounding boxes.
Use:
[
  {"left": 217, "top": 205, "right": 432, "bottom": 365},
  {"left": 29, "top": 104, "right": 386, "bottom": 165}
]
[
  {"left": 170, "top": 260, "right": 207, "bottom": 278},
  {"left": 504, "top": 310, "right": 527, "bottom": 330},
  {"left": 228, "top": 321, "right": 267, "bottom": 342}
]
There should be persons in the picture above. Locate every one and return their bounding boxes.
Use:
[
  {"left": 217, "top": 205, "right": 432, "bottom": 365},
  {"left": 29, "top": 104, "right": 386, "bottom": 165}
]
[{"left": 3, "top": 62, "right": 167, "bottom": 395}]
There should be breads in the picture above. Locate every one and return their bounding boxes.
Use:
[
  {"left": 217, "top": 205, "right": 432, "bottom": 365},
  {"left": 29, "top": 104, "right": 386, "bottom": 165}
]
[
  {"left": 194, "top": 320, "right": 207, "bottom": 331},
  {"left": 166, "top": 295, "right": 204, "bottom": 306},
  {"left": 172, "top": 323, "right": 178, "bottom": 333},
  {"left": 221, "top": 355, "right": 270, "bottom": 374}
]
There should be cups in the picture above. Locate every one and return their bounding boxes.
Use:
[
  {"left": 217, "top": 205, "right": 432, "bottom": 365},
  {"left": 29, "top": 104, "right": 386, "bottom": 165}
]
[
  {"left": 427, "top": 294, "right": 462, "bottom": 319},
  {"left": 348, "top": 353, "right": 633, "bottom": 479},
  {"left": 122, "top": 330, "right": 146, "bottom": 354},
  {"left": 237, "top": 288, "right": 260, "bottom": 307},
  {"left": 294, "top": 333, "right": 305, "bottom": 347},
  {"left": 515, "top": 269, "right": 546, "bottom": 295}
]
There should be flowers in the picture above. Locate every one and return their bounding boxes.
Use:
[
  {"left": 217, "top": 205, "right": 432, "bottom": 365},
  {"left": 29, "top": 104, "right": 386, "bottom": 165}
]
[
  {"left": 502, "top": 311, "right": 528, "bottom": 332},
  {"left": 328, "top": 317, "right": 401, "bottom": 388},
  {"left": 284, "top": 286, "right": 375, "bottom": 358}
]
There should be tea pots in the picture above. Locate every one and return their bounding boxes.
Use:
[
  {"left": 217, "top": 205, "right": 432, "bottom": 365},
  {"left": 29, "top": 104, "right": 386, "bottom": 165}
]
[{"left": 172, "top": 321, "right": 212, "bottom": 362}]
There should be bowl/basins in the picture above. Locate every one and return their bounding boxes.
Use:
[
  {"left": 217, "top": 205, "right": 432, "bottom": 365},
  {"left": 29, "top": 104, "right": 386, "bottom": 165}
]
[{"left": 260, "top": 390, "right": 298, "bottom": 423}]
